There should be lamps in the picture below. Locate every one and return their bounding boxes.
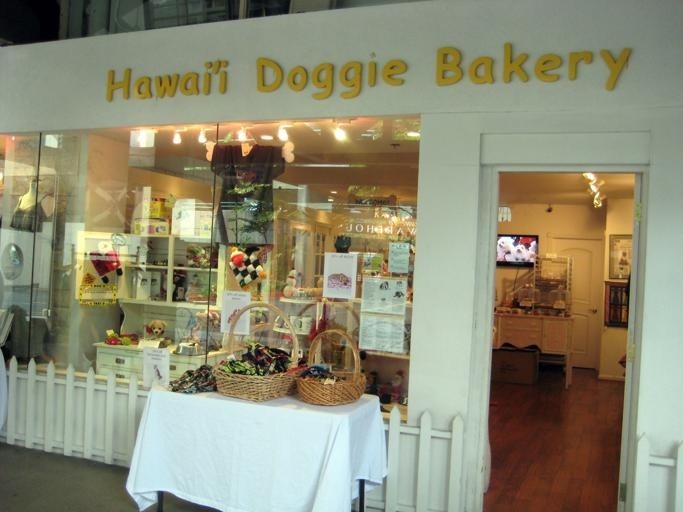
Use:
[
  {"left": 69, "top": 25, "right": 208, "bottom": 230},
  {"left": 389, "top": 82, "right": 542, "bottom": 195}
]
[{"left": 583, "top": 173, "right": 606, "bottom": 209}]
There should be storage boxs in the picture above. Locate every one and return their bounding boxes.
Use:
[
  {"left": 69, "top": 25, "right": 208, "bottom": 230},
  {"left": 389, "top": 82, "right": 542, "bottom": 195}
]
[{"left": 492, "top": 347, "right": 540, "bottom": 387}]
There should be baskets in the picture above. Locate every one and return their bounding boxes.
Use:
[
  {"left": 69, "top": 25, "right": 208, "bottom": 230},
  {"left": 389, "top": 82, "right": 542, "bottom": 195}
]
[
  {"left": 210, "top": 300, "right": 310, "bottom": 404},
  {"left": 292, "top": 328, "right": 367, "bottom": 407}
]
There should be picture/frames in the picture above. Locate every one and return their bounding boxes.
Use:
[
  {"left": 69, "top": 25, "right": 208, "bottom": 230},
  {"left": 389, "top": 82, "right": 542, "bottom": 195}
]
[{"left": 608, "top": 234, "right": 631, "bottom": 281}]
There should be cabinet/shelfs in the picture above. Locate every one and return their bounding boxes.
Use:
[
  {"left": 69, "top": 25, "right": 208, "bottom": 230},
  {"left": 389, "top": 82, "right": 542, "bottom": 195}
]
[
  {"left": 324, "top": 296, "right": 412, "bottom": 359},
  {"left": 71, "top": 230, "right": 270, "bottom": 385},
  {"left": 273, "top": 294, "right": 317, "bottom": 338}
]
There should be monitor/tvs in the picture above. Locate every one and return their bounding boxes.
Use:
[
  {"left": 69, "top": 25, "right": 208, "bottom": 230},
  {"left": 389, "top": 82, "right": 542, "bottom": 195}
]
[{"left": 496, "top": 235, "right": 539, "bottom": 268}]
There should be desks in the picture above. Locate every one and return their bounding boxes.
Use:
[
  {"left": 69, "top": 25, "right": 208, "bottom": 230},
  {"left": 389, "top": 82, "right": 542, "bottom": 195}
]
[
  {"left": 144, "top": 389, "right": 380, "bottom": 512},
  {"left": 492, "top": 314, "right": 575, "bottom": 389}
]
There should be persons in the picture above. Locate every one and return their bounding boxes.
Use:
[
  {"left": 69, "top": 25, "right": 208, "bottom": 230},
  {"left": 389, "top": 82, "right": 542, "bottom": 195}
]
[{"left": 8, "top": 178, "right": 55, "bottom": 233}]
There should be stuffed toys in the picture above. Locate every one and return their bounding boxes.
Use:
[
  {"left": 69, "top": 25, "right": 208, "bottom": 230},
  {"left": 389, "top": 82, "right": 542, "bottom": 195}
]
[
  {"left": 365, "top": 368, "right": 404, "bottom": 404},
  {"left": 187, "top": 243, "right": 218, "bottom": 268},
  {"left": 228, "top": 245, "right": 266, "bottom": 292},
  {"left": 148, "top": 319, "right": 167, "bottom": 338},
  {"left": 281, "top": 268, "right": 301, "bottom": 298}
]
